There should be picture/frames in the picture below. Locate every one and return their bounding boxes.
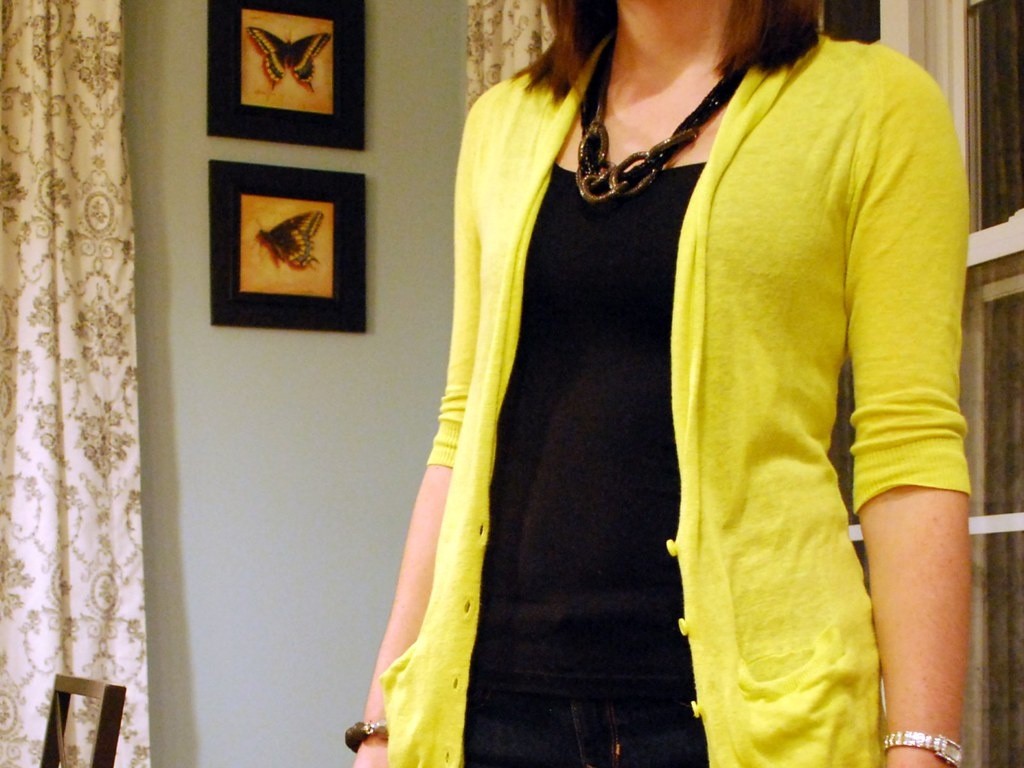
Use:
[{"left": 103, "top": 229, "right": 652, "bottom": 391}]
[
  {"left": 206, "top": 0, "right": 367, "bottom": 152},
  {"left": 208, "top": 159, "right": 366, "bottom": 335}
]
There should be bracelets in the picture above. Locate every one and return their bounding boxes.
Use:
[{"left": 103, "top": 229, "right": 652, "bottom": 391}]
[{"left": 345, "top": 720, "right": 388, "bottom": 753}]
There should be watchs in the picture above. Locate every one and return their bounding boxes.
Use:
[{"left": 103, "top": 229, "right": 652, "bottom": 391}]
[{"left": 883, "top": 730, "right": 962, "bottom": 766}]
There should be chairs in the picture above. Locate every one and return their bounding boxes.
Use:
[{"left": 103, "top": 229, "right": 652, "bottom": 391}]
[{"left": 39, "top": 674, "right": 126, "bottom": 768}]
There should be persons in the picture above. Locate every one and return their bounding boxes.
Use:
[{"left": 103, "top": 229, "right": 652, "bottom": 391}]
[{"left": 352, "top": 0, "right": 972, "bottom": 768}]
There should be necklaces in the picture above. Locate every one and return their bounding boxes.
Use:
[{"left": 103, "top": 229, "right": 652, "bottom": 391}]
[{"left": 577, "top": 79, "right": 700, "bottom": 203}]
